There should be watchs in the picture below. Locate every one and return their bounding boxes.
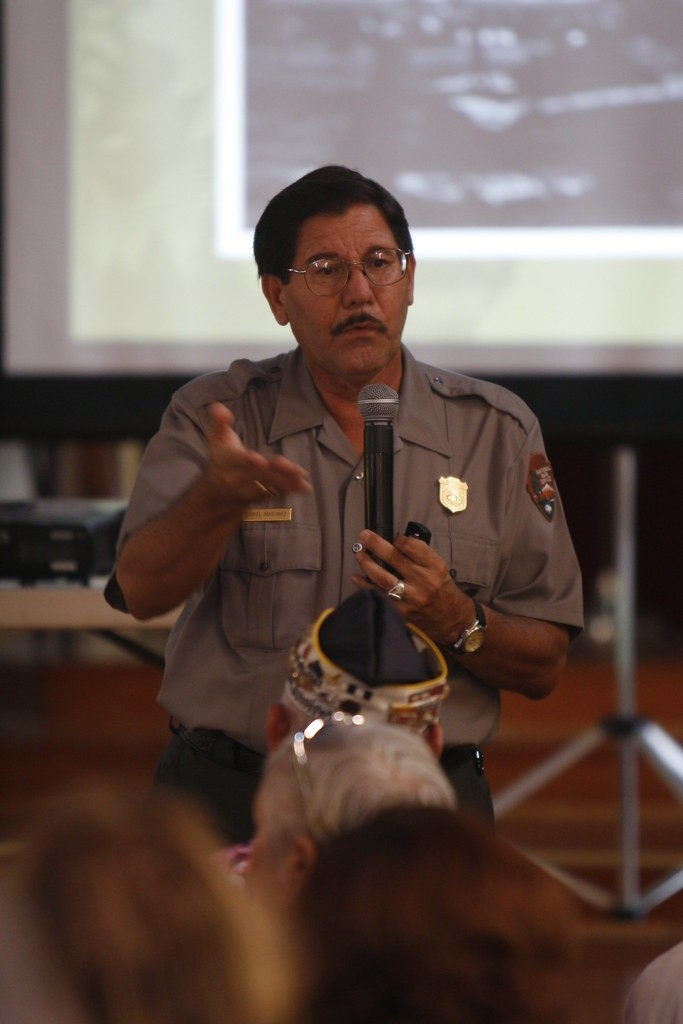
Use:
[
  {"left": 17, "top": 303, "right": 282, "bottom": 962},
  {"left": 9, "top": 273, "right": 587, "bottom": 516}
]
[{"left": 438, "top": 599, "right": 487, "bottom": 656}]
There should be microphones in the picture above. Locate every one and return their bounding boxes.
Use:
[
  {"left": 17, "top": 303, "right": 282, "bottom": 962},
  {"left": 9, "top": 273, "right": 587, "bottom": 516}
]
[{"left": 358, "top": 384, "right": 399, "bottom": 584}]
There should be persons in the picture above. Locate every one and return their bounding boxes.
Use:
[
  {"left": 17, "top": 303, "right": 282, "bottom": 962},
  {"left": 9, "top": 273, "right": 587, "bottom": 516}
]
[
  {"left": 0, "top": 585, "right": 683, "bottom": 1024},
  {"left": 99, "top": 161, "right": 587, "bottom": 859}
]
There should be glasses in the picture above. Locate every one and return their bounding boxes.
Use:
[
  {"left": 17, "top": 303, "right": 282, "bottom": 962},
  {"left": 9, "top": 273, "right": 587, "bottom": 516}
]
[
  {"left": 291, "top": 711, "right": 367, "bottom": 837},
  {"left": 285, "top": 247, "right": 412, "bottom": 297}
]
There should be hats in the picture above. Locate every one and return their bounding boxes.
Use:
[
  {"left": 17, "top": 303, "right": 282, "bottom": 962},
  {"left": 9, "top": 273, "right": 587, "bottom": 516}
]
[{"left": 279, "top": 588, "right": 464, "bottom": 740}]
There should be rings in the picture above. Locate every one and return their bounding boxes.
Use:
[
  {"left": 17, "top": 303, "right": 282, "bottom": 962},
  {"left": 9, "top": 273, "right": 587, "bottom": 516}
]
[
  {"left": 387, "top": 580, "right": 404, "bottom": 602},
  {"left": 255, "top": 480, "right": 267, "bottom": 495}
]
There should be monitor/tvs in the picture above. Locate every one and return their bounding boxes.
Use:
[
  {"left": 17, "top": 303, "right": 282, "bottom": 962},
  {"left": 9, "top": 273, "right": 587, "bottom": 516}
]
[{"left": 0, "top": 500, "right": 129, "bottom": 582}]
